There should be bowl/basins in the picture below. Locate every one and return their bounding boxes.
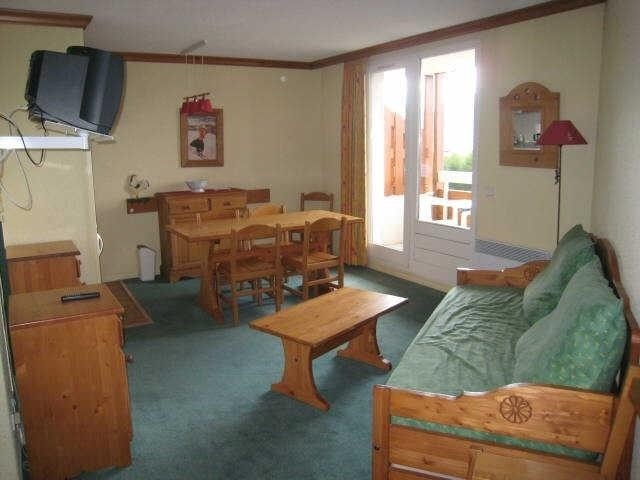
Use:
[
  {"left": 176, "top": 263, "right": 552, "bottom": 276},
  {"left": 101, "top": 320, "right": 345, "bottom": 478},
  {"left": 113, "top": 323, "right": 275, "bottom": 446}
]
[{"left": 183, "top": 179, "right": 210, "bottom": 194}]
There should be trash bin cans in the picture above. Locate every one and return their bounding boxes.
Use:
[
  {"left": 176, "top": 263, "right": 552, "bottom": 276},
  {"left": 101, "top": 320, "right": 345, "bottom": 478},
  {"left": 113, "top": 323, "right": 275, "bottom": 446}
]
[{"left": 136, "top": 245, "right": 157, "bottom": 282}]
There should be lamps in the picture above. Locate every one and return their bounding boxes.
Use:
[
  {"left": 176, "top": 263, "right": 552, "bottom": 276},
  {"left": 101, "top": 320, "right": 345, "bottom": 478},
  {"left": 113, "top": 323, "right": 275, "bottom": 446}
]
[
  {"left": 533, "top": 119, "right": 587, "bottom": 249},
  {"left": 178, "top": 40, "right": 214, "bottom": 117}
]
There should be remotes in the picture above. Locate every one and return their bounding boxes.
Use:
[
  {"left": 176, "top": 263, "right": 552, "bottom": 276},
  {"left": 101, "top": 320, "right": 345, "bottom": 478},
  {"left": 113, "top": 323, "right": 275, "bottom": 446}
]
[{"left": 61, "top": 292, "right": 100, "bottom": 301}]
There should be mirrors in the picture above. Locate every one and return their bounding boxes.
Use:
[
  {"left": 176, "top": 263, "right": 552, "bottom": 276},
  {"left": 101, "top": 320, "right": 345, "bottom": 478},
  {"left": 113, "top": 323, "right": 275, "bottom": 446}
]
[{"left": 497, "top": 83, "right": 561, "bottom": 170}]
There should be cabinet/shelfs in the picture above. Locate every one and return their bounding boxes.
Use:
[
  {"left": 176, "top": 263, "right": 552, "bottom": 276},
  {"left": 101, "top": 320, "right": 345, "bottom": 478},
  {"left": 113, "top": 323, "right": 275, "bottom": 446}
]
[
  {"left": 7, "top": 283, "right": 133, "bottom": 479},
  {"left": 6, "top": 237, "right": 83, "bottom": 293}
]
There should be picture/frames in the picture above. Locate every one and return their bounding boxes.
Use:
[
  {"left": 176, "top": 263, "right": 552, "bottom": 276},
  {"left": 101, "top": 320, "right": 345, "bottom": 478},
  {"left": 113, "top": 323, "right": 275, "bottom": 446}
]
[{"left": 179, "top": 108, "right": 223, "bottom": 167}]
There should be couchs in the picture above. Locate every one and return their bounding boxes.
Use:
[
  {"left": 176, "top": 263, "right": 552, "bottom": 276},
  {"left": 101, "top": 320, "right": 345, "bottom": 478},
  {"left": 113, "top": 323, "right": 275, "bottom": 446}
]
[{"left": 371, "top": 234, "right": 639, "bottom": 479}]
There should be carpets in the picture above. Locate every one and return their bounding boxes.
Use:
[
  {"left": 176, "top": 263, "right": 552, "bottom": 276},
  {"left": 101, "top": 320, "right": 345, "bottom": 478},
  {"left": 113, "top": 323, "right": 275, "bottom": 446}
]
[{"left": 105, "top": 275, "right": 156, "bottom": 330}]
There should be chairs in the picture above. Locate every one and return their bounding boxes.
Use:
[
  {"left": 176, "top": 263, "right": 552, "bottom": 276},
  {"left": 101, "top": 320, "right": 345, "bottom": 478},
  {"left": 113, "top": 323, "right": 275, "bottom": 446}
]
[{"left": 160, "top": 188, "right": 365, "bottom": 323}]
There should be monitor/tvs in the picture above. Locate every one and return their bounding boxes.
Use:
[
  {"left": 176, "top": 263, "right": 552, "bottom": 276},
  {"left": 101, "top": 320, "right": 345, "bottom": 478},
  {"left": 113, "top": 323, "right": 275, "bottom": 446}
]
[{"left": 24, "top": 46, "right": 124, "bottom": 135}]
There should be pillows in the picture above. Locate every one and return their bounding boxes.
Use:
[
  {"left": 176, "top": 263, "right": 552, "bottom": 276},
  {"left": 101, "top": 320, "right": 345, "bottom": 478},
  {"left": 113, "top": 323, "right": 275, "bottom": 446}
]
[
  {"left": 523, "top": 222, "right": 593, "bottom": 325},
  {"left": 511, "top": 256, "right": 628, "bottom": 422}
]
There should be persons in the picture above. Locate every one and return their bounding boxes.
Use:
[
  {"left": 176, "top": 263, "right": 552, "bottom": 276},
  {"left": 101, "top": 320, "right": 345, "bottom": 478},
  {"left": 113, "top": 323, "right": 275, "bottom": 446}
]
[{"left": 190, "top": 123, "right": 209, "bottom": 156}]
